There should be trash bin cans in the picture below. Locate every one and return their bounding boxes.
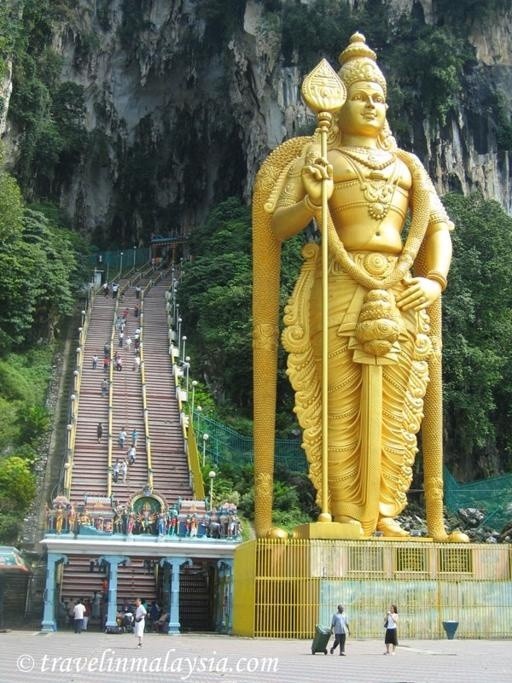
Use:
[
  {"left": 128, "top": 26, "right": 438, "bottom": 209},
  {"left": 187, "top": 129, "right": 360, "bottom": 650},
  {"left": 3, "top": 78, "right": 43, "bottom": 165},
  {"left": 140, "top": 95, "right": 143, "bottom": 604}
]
[{"left": 312, "top": 624, "right": 332, "bottom": 655}]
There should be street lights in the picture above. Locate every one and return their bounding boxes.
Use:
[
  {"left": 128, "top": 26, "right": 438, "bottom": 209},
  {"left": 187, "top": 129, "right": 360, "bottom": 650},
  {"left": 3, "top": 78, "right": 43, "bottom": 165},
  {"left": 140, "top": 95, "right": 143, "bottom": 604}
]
[
  {"left": 208, "top": 471, "right": 215, "bottom": 512},
  {"left": 177, "top": 317, "right": 182, "bottom": 350},
  {"left": 186, "top": 356, "right": 191, "bottom": 392},
  {"left": 202, "top": 434, "right": 208, "bottom": 467},
  {"left": 133, "top": 246, "right": 136, "bottom": 266},
  {"left": 120, "top": 252, "right": 124, "bottom": 274},
  {"left": 182, "top": 335, "right": 187, "bottom": 362},
  {"left": 171, "top": 257, "right": 183, "bottom": 331},
  {"left": 197, "top": 406, "right": 202, "bottom": 439},
  {"left": 191, "top": 380, "right": 197, "bottom": 418},
  {"left": 63, "top": 284, "right": 95, "bottom": 489}
]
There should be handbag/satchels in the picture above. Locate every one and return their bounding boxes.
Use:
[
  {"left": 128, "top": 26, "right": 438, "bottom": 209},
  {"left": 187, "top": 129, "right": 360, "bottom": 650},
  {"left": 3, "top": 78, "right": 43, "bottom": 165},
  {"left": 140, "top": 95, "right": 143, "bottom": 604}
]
[
  {"left": 135, "top": 618, "right": 142, "bottom": 622},
  {"left": 384, "top": 620, "right": 388, "bottom": 628}
]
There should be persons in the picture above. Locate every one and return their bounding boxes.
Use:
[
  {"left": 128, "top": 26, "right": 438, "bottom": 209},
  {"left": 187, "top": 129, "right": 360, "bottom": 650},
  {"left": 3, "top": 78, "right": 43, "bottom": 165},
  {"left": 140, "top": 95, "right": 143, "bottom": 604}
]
[
  {"left": 121, "top": 458, "right": 129, "bottom": 483},
  {"left": 101, "top": 378, "right": 109, "bottom": 397},
  {"left": 127, "top": 445, "right": 137, "bottom": 466},
  {"left": 66, "top": 559, "right": 169, "bottom": 650},
  {"left": 92, "top": 255, "right": 165, "bottom": 373},
  {"left": 131, "top": 429, "right": 139, "bottom": 448},
  {"left": 55, "top": 503, "right": 237, "bottom": 540},
  {"left": 117, "top": 427, "right": 128, "bottom": 448},
  {"left": 97, "top": 422, "right": 103, "bottom": 444},
  {"left": 384, "top": 604, "right": 398, "bottom": 656},
  {"left": 330, "top": 604, "right": 351, "bottom": 656},
  {"left": 112, "top": 457, "right": 121, "bottom": 483},
  {"left": 254, "top": 29, "right": 471, "bottom": 544}
]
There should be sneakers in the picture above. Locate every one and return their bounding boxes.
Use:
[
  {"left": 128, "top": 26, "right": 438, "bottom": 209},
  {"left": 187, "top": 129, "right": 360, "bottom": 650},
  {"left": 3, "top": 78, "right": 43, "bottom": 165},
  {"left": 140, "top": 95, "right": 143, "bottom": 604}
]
[
  {"left": 137, "top": 642, "right": 143, "bottom": 648},
  {"left": 340, "top": 654, "right": 346, "bottom": 656},
  {"left": 384, "top": 651, "right": 396, "bottom": 656},
  {"left": 330, "top": 646, "right": 334, "bottom": 654}
]
